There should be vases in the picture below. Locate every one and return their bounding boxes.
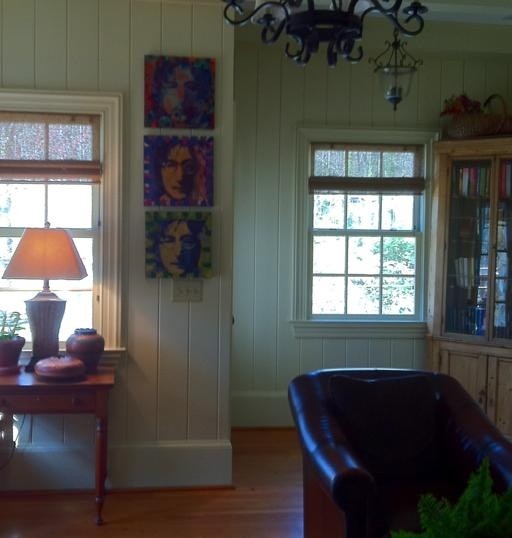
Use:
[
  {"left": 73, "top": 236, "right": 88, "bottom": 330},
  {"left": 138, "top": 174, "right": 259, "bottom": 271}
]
[{"left": 63, "top": 327, "right": 105, "bottom": 371}]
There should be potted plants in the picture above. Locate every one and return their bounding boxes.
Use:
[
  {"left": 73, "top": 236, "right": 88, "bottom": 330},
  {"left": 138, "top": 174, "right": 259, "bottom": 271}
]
[{"left": 0, "top": 306, "right": 32, "bottom": 372}]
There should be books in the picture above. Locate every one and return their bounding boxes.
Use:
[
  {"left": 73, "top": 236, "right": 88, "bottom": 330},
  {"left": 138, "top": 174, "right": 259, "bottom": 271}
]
[{"left": 445, "top": 159, "right": 511, "bottom": 338}]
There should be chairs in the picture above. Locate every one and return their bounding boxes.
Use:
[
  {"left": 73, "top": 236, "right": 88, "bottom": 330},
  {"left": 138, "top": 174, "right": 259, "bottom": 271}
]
[{"left": 285, "top": 362, "right": 512, "bottom": 537}]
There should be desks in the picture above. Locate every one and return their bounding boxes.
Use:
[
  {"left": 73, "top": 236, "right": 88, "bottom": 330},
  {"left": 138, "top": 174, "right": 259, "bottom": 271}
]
[{"left": 0, "top": 361, "right": 118, "bottom": 528}]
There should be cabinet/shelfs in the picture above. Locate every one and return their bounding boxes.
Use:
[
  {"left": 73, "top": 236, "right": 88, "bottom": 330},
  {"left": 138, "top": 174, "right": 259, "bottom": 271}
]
[
  {"left": 423, "top": 134, "right": 512, "bottom": 355},
  {"left": 423, "top": 337, "right": 512, "bottom": 445}
]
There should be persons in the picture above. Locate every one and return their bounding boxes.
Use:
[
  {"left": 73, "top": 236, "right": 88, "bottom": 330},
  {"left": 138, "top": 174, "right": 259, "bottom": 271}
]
[
  {"left": 156, "top": 142, "right": 205, "bottom": 206},
  {"left": 154, "top": 220, "right": 206, "bottom": 278},
  {"left": 153, "top": 59, "right": 210, "bottom": 127}
]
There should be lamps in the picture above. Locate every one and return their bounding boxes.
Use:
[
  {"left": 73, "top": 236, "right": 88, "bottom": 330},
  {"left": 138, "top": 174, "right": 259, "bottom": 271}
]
[
  {"left": 220, "top": 0, "right": 429, "bottom": 112},
  {"left": 0, "top": 220, "right": 89, "bottom": 372}
]
